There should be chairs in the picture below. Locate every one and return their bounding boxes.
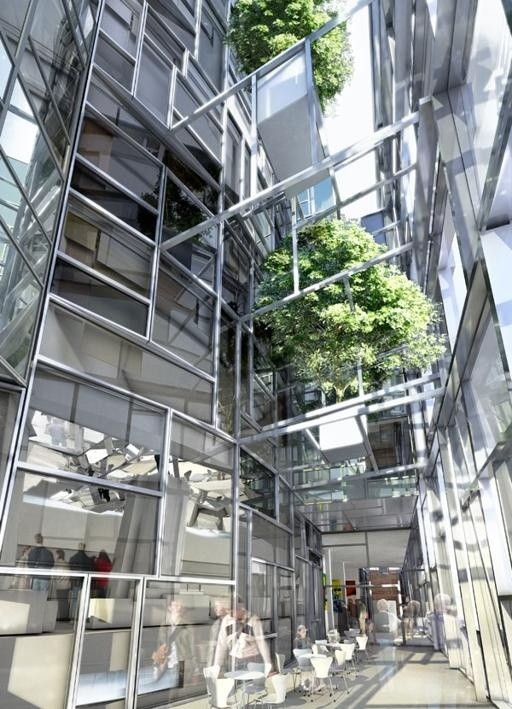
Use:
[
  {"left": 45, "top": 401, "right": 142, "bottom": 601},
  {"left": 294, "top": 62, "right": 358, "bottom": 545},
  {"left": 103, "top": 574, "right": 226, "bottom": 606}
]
[{"left": 203, "top": 627, "right": 372, "bottom": 709}]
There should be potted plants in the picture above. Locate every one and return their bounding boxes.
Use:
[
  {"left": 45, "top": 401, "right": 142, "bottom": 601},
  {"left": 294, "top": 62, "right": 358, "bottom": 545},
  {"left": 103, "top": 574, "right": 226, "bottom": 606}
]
[
  {"left": 224, "top": 1, "right": 355, "bottom": 201},
  {"left": 245, "top": 212, "right": 450, "bottom": 467}
]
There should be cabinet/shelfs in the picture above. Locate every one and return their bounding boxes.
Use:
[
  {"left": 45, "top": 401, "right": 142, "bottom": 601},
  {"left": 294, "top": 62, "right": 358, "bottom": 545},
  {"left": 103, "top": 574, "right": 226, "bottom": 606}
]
[{"left": 178, "top": 559, "right": 234, "bottom": 579}]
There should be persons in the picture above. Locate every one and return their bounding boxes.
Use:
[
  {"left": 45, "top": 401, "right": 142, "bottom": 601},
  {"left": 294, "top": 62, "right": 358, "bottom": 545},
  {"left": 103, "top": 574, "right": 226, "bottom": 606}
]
[
  {"left": 94, "top": 549, "right": 113, "bottom": 597},
  {"left": 27, "top": 532, "right": 55, "bottom": 591},
  {"left": 68, "top": 541, "right": 95, "bottom": 623},
  {"left": 151, "top": 593, "right": 462, "bottom": 707},
  {"left": 51, "top": 547, "right": 72, "bottom": 622},
  {"left": 12, "top": 545, "right": 32, "bottom": 589}
]
